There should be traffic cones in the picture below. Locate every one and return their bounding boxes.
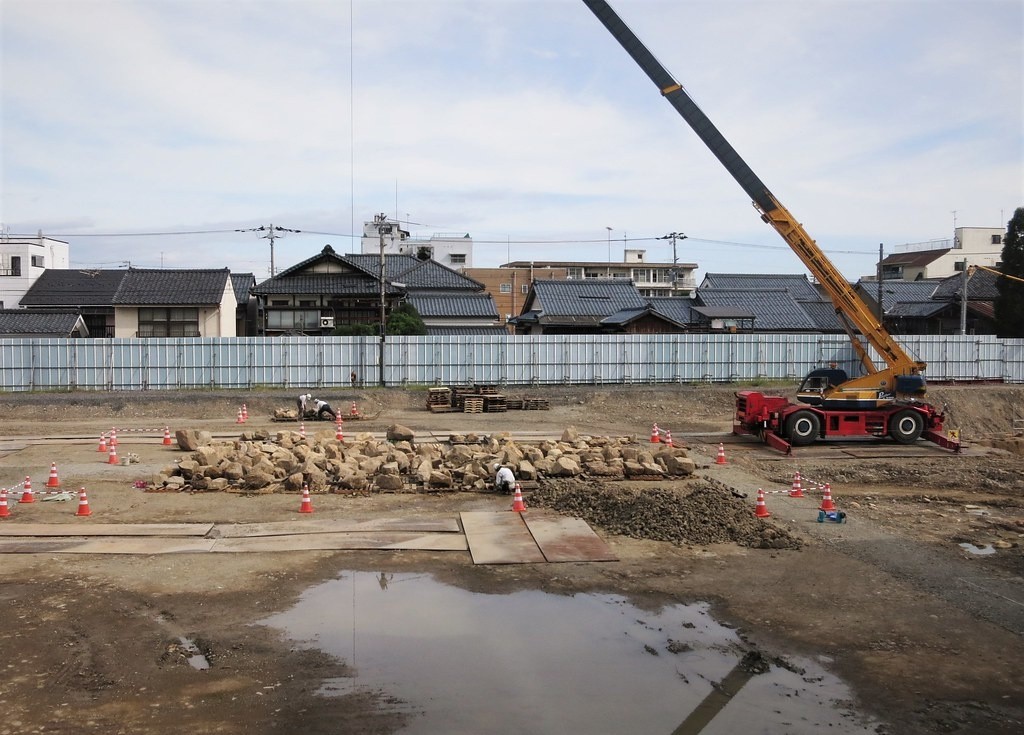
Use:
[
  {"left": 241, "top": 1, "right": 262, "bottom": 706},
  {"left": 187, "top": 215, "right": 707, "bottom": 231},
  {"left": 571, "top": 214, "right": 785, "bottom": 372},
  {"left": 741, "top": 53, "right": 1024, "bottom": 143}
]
[
  {"left": 335, "top": 423, "right": 343, "bottom": 439},
  {"left": 161, "top": 426, "right": 173, "bottom": 445},
  {"left": 75, "top": 487, "right": 92, "bottom": 516},
  {"left": 818, "top": 483, "right": 836, "bottom": 511},
  {"left": 351, "top": 401, "right": 360, "bottom": 414},
  {"left": 788, "top": 472, "right": 804, "bottom": 497},
  {"left": 714, "top": 442, "right": 727, "bottom": 464},
  {"left": 45, "top": 463, "right": 60, "bottom": 486},
  {"left": 665, "top": 429, "right": 674, "bottom": 449},
  {"left": 298, "top": 485, "right": 314, "bottom": 513},
  {"left": 0, "top": 476, "right": 35, "bottom": 516},
  {"left": 299, "top": 423, "right": 306, "bottom": 436},
  {"left": 334, "top": 407, "right": 344, "bottom": 424},
  {"left": 235, "top": 407, "right": 245, "bottom": 423},
  {"left": 242, "top": 404, "right": 249, "bottom": 419},
  {"left": 753, "top": 488, "right": 770, "bottom": 516},
  {"left": 97, "top": 427, "right": 120, "bottom": 464},
  {"left": 650, "top": 423, "right": 660, "bottom": 443},
  {"left": 511, "top": 483, "right": 525, "bottom": 511}
]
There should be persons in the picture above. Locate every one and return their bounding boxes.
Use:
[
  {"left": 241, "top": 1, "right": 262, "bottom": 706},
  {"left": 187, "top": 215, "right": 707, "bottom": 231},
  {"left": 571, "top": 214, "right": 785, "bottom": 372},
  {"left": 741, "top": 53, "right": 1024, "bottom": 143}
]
[
  {"left": 296, "top": 394, "right": 337, "bottom": 421},
  {"left": 493, "top": 464, "right": 515, "bottom": 495}
]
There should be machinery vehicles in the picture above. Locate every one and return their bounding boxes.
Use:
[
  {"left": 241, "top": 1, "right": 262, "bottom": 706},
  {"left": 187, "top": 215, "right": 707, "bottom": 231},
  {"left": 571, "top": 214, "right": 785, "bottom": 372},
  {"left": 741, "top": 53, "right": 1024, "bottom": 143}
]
[{"left": 584, "top": 0, "right": 963, "bottom": 455}]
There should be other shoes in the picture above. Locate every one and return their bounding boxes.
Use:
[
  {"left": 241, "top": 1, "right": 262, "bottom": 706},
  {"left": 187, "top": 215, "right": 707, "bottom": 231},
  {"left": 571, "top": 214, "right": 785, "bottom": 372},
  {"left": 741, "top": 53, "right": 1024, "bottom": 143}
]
[{"left": 502, "top": 491, "right": 512, "bottom": 495}]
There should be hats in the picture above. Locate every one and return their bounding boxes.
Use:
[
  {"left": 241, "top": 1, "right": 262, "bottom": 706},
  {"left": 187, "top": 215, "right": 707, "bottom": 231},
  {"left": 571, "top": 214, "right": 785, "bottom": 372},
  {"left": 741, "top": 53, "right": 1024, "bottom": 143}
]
[
  {"left": 307, "top": 394, "right": 312, "bottom": 400},
  {"left": 493, "top": 463, "right": 500, "bottom": 470},
  {"left": 313, "top": 399, "right": 319, "bottom": 403}
]
